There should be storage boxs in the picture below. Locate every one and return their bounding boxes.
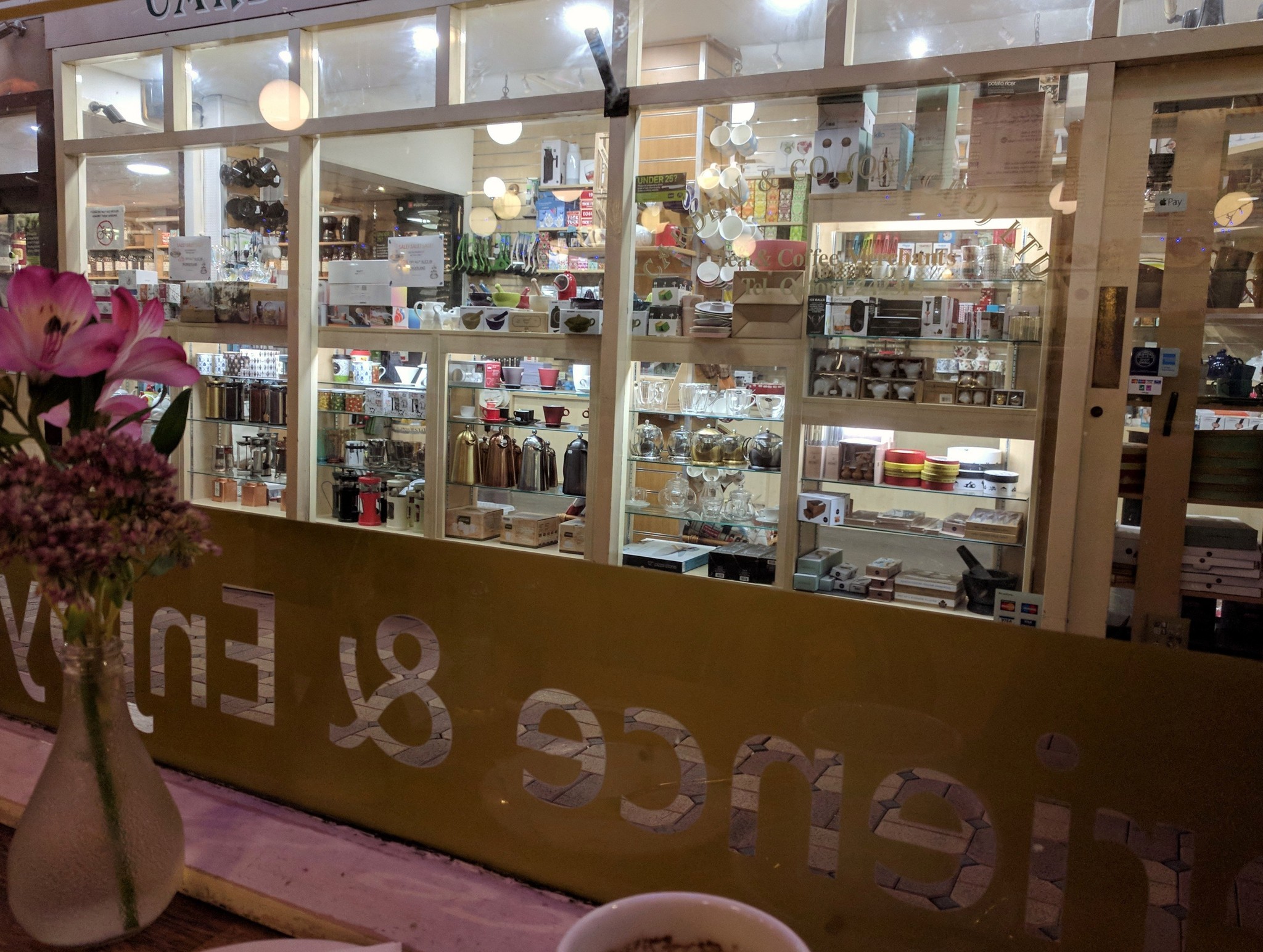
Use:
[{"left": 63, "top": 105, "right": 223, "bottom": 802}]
[
  {"left": 445, "top": 502, "right": 586, "bottom": 555},
  {"left": 708, "top": 541, "right": 778, "bottom": 586},
  {"left": 623, "top": 538, "right": 717, "bottom": 574},
  {"left": 91, "top": 259, "right": 808, "bottom": 335},
  {"left": 1120, "top": 143, "right": 1262, "bottom": 658},
  {"left": 804, "top": 294, "right": 1041, "bottom": 406},
  {"left": 793, "top": 440, "right": 1023, "bottom": 611},
  {"left": 366, "top": 387, "right": 427, "bottom": 417},
  {"left": 127, "top": 232, "right": 169, "bottom": 246},
  {"left": 729, "top": 71, "right": 1070, "bottom": 257}
]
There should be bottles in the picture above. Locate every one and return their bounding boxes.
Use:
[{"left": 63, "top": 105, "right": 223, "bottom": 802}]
[
  {"left": 320, "top": 470, "right": 425, "bottom": 531},
  {"left": 550, "top": 306, "right": 560, "bottom": 328}
]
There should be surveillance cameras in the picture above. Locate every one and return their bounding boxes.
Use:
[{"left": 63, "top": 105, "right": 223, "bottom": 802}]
[{"left": 102, "top": 104, "right": 126, "bottom": 124}]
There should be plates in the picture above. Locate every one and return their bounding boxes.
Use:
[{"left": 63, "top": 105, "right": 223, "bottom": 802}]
[
  {"left": 625, "top": 500, "right": 650, "bottom": 509},
  {"left": 452, "top": 415, "right": 589, "bottom": 428},
  {"left": 755, "top": 517, "right": 778, "bottom": 524},
  {"left": 689, "top": 300, "right": 733, "bottom": 338}
]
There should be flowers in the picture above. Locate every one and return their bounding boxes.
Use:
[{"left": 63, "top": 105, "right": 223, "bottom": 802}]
[{"left": 0, "top": 264, "right": 224, "bottom": 934}]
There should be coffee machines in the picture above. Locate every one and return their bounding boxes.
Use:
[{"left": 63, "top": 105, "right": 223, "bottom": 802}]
[
  {"left": 319, "top": 216, "right": 337, "bottom": 242},
  {"left": 322, "top": 245, "right": 367, "bottom": 261},
  {"left": 339, "top": 215, "right": 360, "bottom": 242}
]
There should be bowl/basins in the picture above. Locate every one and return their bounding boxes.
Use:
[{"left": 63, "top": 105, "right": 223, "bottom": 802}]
[
  {"left": 749, "top": 239, "right": 806, "bottom": 271},
  {"left": 467, "top": 293, "right": 556, "bottom": 312},
  {"left": 485, "top": 314, "right": 504, "bottom": 329},
  {"left": 964, "top": 569, "right": 1019, "bottom": 613},
  {"left": 462, "top": 313, "right": 481, "bottom": 330}
]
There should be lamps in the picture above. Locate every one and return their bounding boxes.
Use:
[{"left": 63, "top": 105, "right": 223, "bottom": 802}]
[{"left": 486, "top": 74, "right": 523, "bottom": 145}]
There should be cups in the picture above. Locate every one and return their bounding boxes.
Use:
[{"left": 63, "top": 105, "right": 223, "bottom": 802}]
[
  {"left": 1207, "top": 349, "right": 1256, "bottom": 397},
  {"left": 654, "top": 224, "right": 682, "bottom": 247},
  {"left": 815, "top": 247, "right": 1039, "bottom": 280},
  {"left": 220, "top": 156, "right": 287, "bottom": 233},
  {"left": 626, "top": 486, "right": 647, "bottom": 504},
  {"left": 682, "top": 295, "right": 704, "bottom": 336},
  {"left": 686, "top": 465, "right": 746, "bottom": 492},
  {"left": 1210, "top": 246, "right": 1262, "bottom": 310},
  {"left": 758, "top": 507, "right": 779, "bottom": 522},
  {"left": 327, "top": 351, "right": 425, "bottom": 473},
  {"left": 459, "top": 367, "right": 590, "bottom": 424},
  {"left": 636, "top": 380, "right": 785, "bottom": 420},
  {"left": 195, "top": 353, "right": 288, "bottom": 481},
  {"left": 697, "top": 120, "right": 764, "bottom": 290}
]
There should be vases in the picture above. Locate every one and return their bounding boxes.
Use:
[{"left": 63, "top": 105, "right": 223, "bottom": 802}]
[{"left": 8, "top": 636, "right": 187, "bottom": 949}]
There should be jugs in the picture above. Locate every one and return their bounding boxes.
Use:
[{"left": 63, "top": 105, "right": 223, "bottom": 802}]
[
  {"left": 658, "top": 473, "right": 756, "bottom": 522},
  {"left": 564, "top": 315, "right": 595, "bottom": 332},
  {"left": 413, "top": 300, "right": 460, "bottom": 330},
  {"left": 448, "top": 426, "right": 587, "bottom": 495}
]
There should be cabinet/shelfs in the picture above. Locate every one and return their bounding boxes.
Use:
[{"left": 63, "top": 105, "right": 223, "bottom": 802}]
[
  {"left": 188, "top": 370, "right": 291, "bottom": 520},
  {"left": 802, "top": 276, "right": 1044, "bottom": 411},
  {"left": 89, "top": 240, "right": 361, "bottom": 284},
  {"left": 622, "top": 409, "right": 784, "bottom": 587},
  {"left": 790, "top": 477, "right": 1030, "bottom": 621},
  {"left": 1108, "top": 103, "right": 1263, "bottom": 653},
  {"left": 446, "top": 381, "right": 592, "bottom": 558},
  {"left": 318, "top": 380, "right": 428, "bottom": 535},
  {"left": 537, "top": 182, "right": 603, "bottom": 275}
]
